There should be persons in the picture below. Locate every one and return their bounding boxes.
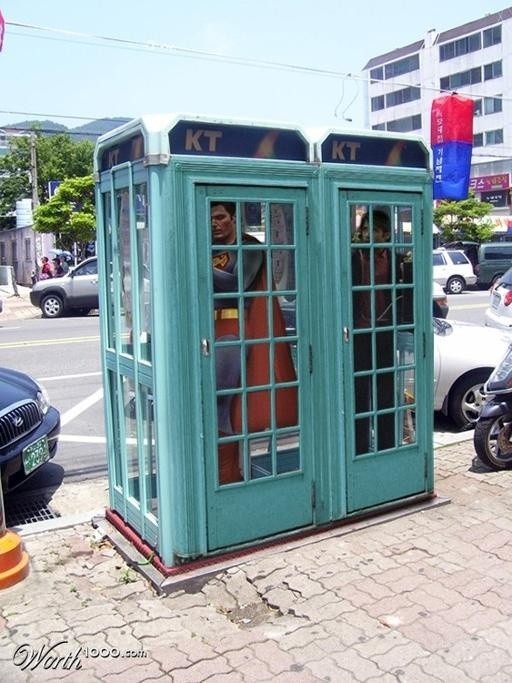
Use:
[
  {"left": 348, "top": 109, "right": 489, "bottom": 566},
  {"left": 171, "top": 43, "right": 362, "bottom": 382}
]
[
  {"left": 208, "top": 200, "right": 266, "bottom": 483},
  {"left": 53, "top": 257, "right": 65, "bottom": 277},
  {"left": 349, "top": 209, "right": 410, "bottom": 455},
  {"left": 41, "top": 256, "right": 50, "bottom": 279},
  {"left": 60, "top": 255, "right": 70, "bottom": 273}
]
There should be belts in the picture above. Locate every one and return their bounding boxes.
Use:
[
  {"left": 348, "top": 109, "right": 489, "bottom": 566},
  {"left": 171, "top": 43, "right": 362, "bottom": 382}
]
[{"left": 214, "top": 309, "right": 248, "bottom": 320}]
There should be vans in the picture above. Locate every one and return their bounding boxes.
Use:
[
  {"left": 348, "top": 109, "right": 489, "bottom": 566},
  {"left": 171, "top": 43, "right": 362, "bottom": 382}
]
[{"left": 442, "top": 240, "right": 511, "bottom": 287}]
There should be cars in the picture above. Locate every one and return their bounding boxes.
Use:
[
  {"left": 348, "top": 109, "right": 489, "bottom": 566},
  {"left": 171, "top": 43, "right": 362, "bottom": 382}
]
[
  {"left": 1, "top": 366, "right": 61, "bottom": 493},
  {"left": 398, "top": 315, "right": 511, "bottom": 429},
  {"left": 432, "top": 282, "right": 448, "bottom": 318},
  {"left": 432, "top": 247, "right": 477, "bottom": 292},
  {"left": 30, "top": 255, "right": 113, "bottom": 318},
  {"left": 483, "top": 265, "right": 511, "bottom": 330}
]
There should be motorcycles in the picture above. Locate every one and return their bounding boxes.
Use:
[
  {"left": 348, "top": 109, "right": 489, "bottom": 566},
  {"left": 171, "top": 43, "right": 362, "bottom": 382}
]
[{"left": 473, "top": 343, "right": 511, "bottom": 470}]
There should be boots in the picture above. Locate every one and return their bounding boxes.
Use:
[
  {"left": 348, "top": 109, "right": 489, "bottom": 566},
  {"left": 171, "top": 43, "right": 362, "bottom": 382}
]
[{"left": 219, "top": 431, "right": 243, "bottom": 485}]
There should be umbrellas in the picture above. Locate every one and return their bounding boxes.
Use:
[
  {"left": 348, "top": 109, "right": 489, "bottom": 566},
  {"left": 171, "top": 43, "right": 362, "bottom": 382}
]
[{"left": 49, "top": 249, "right": 76, "bottom": 257}]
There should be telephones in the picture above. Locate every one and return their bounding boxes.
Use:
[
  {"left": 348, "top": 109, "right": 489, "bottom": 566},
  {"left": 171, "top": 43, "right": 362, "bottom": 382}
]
[{"left": 355, "top": 226, "right": 365, "bottom": 248}]
